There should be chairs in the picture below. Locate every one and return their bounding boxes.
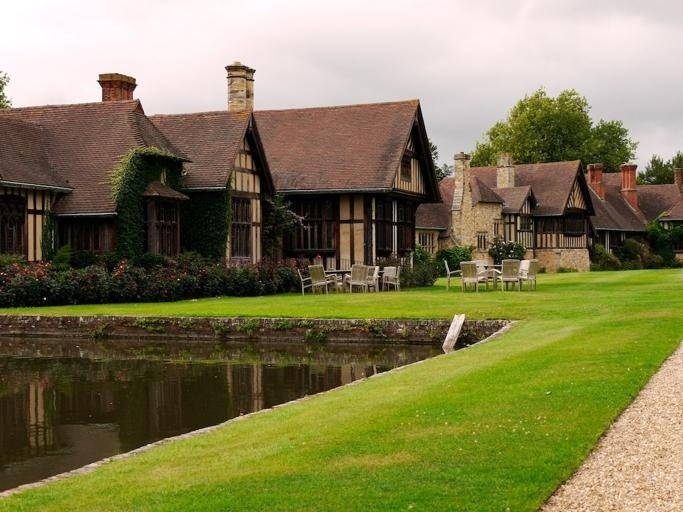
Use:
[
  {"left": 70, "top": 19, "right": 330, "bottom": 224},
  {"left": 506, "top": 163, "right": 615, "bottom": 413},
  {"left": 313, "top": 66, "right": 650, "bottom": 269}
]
[
  {"left": 296, "top": 263, "right": 405, "bottom": 295},
  {"left": 443, "top": 256, "right": 538, "bottom": 294}
]
